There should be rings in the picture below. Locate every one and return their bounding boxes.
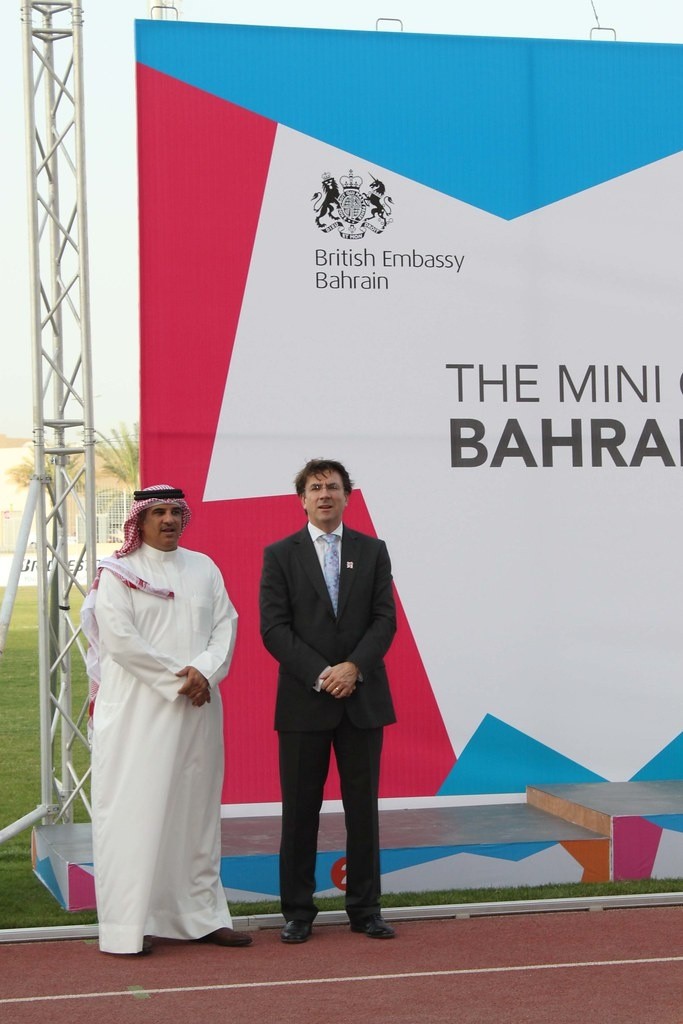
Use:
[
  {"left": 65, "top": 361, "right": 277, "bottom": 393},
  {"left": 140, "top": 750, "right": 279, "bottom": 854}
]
[{"left": 336, "top": 688, "right": 341, "bottom": 692}]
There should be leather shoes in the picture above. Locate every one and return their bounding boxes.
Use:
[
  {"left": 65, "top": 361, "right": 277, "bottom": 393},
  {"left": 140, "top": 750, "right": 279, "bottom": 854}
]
[
  {"left": 280, "top": 919, "right": 312, "bottom": 942},
  {"left": 350, "top": 914, "right": 395, "bottom": 938}
]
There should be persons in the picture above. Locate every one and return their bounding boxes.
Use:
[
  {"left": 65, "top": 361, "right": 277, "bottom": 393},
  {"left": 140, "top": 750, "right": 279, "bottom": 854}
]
[
  {"left": 259, "top": 460, "right": 397, "bottom": 944},
  {"left": 79, "top": 484, "right": 253, "bottom": 956}
]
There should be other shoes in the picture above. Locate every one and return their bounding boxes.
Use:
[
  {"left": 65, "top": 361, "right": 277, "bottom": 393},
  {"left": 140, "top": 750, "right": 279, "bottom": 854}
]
[
  {"left": 132, "top": 936, "right": 153, "bottom": 955},
  {"left": 188, "top": 928, "right": 252, "bottom": 946}
]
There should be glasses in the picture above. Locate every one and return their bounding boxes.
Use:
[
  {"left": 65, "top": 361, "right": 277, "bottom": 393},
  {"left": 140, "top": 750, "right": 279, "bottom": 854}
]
[{"left": 304, "top": 483, "right": 345, "bottom": 490}]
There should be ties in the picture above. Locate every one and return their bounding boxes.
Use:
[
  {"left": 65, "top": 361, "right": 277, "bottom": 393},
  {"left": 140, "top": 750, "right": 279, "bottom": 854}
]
[{"left": 320, "top": 534, "right": 339, "bottom": 617}]
